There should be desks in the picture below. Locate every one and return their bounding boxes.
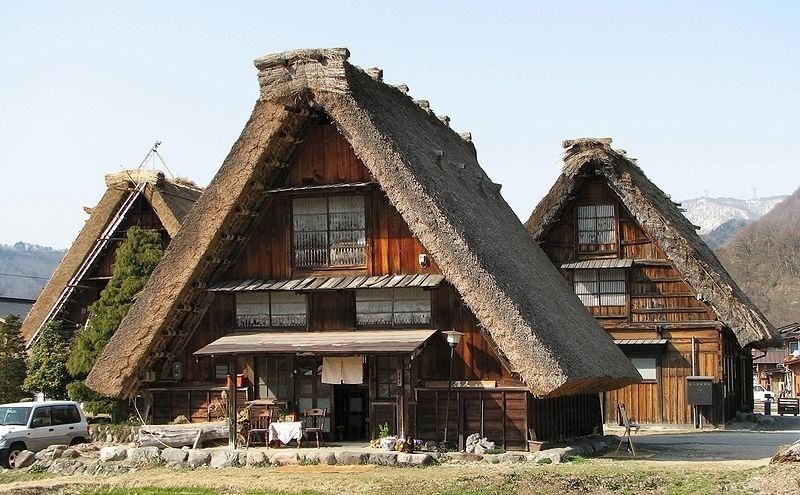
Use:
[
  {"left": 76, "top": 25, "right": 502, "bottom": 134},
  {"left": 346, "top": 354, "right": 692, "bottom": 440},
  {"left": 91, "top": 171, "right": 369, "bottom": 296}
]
[{"left": 267, "top": 421, "right": 303, "bottom": 448}]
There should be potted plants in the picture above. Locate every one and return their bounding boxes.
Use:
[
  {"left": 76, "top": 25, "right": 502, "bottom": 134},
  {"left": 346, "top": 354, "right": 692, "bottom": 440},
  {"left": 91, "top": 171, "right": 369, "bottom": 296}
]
[{"left": 377, "top": 422, "right": 396, "bottom": 451}]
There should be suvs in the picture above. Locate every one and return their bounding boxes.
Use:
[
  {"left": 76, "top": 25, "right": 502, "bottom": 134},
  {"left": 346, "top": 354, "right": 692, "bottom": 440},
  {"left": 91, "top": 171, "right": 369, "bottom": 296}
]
[
  {"left": 753, "top": 383, "right": 776, "bottom": 404},
  {"left": 0, "top": 399, "right": 93, "bottom": 471}
]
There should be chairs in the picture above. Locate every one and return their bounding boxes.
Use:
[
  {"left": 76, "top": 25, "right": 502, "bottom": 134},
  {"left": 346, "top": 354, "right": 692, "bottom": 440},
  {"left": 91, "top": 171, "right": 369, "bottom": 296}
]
[
  {"left": 247, "top": 409, "right": 274, "bottom": 447},
  {"left": 296, "top": 407, "right": 327, "bottom": 448}
]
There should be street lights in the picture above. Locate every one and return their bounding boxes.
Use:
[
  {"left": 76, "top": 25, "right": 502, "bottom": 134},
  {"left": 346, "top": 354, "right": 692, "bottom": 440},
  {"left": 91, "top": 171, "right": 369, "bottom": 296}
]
[{"left": 440, "top": 328, "right": 467, "bottom": 444}]
